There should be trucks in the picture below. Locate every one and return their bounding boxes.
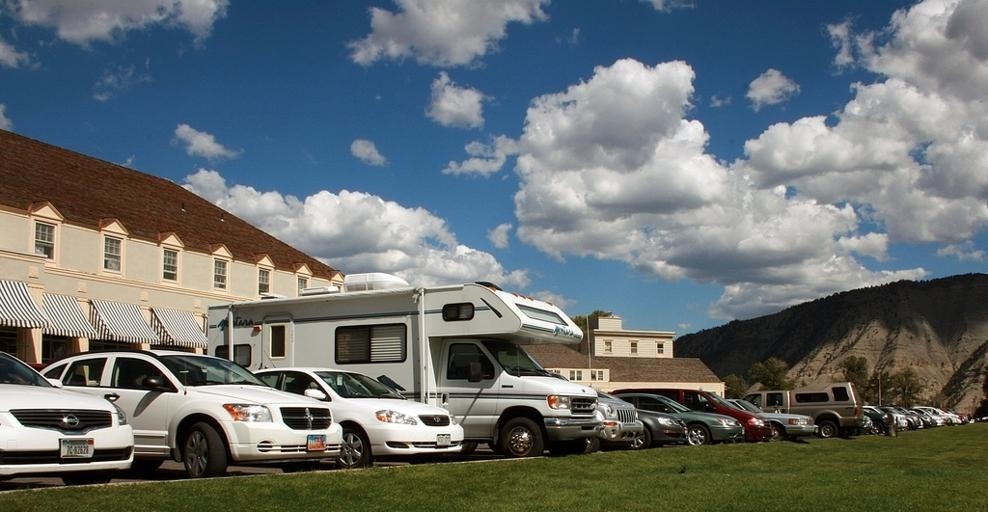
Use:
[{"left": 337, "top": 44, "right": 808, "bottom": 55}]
[
  {"left": 742, "top": 381, "right": 863, "bottom": 438},
  {"left": 207, "top": 272, "right": 599, "bottom": 457}
]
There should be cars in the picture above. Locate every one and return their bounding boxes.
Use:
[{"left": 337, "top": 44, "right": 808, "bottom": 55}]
[
  {"left": 251, "top": 367, "right": 465, "bottom": 468},
  {"left": 626, "top": 409, "right": 688, "bottom": 451},
  {"left": 699, "top": 398, "right": 819, "bottom": 440},
  {"left": 573, "top": 389, "right": 645, "bottom": 454},
  {"left": 39, "top": 348, "right": 344, "bottom": 477},
  {"left": 855, "top": 404, "right": 970, "bottom": 435},
  {"left": 609, "top": 388, "right": 771, "bottom": 443},
  {"left": 0, "top": 350, "right": 135, "bottom": 484},
  {"left": 612, "top": 393, "right": 746, "bottom": 446}
]
